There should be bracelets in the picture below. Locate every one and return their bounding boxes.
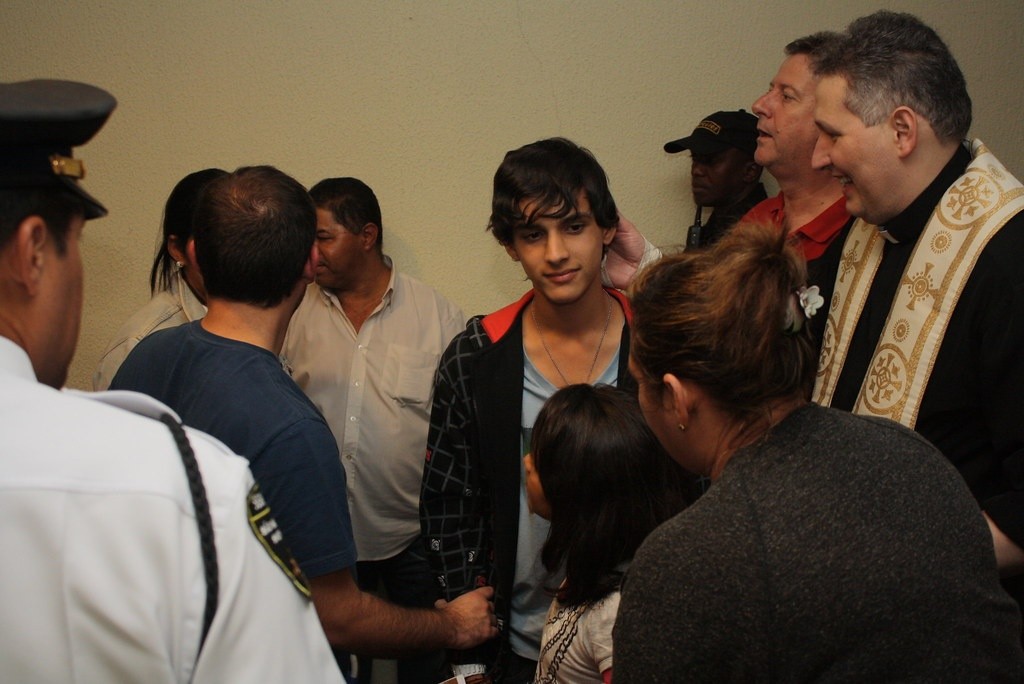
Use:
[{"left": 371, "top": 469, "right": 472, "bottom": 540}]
[{"left": 453, "top": 665, "right": 485, "bottom": 678}]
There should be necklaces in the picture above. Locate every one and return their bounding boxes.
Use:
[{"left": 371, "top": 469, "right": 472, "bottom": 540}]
[{"left": 531, "top": 289, "right": 612, "bottom": 387}]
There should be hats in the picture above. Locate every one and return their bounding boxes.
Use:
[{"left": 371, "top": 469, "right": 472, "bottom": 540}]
[
  {"left": 0, "top": 79, "right": 116, "bottom": 217},
  {"left": 664, "top": 109, "right": 759, "bottom": 155}
]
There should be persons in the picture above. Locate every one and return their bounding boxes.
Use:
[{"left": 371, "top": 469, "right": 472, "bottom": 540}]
[
  {"left": 92, "top": 168, "right": 227, "bottom": 393},
  {"left": 0, "top": 79, "right": 346, "bottom": 684},
  {"left": 598, "top": 30, "right": 853, "bottom": 264},
  {"left": 600, "top": 11, "right": 1024, "bottom": 572},
  {"left": 665, "top": 108, "right": 768, "bottom": 251},
  {"left": 420, "top": 136, "right": 635, "bottom": 684},
  {"left": 524, "top": 383, "right": 689, "bottom": 682},
  {"left": 280, "top": 176, "right": 471, "bottom": 684},
  {"left": 611, "top": 234, "right": 1024, "bottom": 684},
  {"left": 105, "top": 165, "right": 497, "bottom": 654}
]
